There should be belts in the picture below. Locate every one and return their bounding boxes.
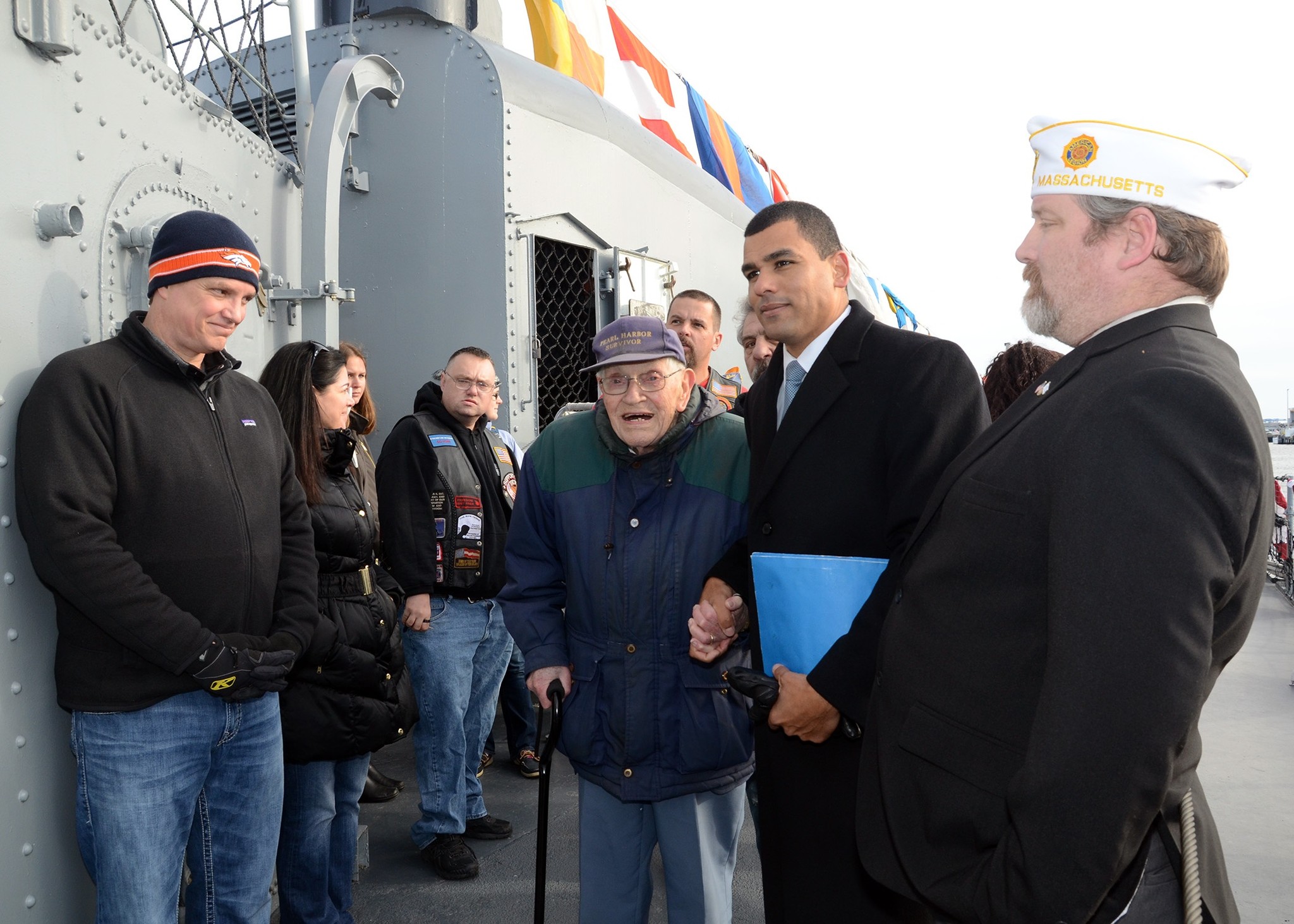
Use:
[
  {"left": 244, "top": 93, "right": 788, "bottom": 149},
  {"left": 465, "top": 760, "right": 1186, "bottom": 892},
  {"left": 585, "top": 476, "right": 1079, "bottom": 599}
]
[{"left": 431, "top": 590, "right": 490, "bottom": 603}]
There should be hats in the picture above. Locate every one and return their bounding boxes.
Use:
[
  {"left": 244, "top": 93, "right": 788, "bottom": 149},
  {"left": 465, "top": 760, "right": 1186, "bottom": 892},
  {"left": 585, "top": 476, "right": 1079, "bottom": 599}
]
[
  {"left": 579, "top": 316, "right": 686, "bottom": 372},
  {"left": 148, "top": 210, "right": 260, "bottom": 299},
  {"left": 1027, "top": 115, "right": 1252, "bottom": 225},
  {"left": 493, "top": 374, "right": 502, "bottom": 388}
]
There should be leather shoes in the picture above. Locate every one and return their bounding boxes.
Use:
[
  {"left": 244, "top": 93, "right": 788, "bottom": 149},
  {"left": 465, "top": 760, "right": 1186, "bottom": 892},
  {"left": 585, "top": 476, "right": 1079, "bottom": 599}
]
[{"left": 358, "top": 761, "right": 405, "bottom": 803}]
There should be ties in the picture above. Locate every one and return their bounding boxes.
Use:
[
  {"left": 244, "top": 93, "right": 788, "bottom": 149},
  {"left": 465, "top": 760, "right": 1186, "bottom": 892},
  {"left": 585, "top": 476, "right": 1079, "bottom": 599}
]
[{"left": 781, "top": 360, "right": 806, "bottom": 422}]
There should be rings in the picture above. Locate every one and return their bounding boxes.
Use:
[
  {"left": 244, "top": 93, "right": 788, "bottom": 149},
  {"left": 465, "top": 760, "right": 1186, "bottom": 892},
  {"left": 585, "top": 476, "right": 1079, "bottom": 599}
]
[
  {"left": 710, "top": 633, "right": 714, "bottom": 645},
  {"left": 423, "top": 619, "right": 431, "bottom": 623}
]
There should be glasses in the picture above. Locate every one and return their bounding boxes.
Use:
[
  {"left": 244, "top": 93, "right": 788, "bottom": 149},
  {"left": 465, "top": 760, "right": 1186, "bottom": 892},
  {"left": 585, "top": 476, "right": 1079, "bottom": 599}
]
[
  {"left": 599, "top": 368, "right": 682, "bottom": 395},
  {"left": 491, "top": 392, "right": 499, "bottom": 399},
  {"left": 311, "top": 339, "right": 329, "bottom": 371},
  {"left": 446, "top": 371, "right": 494, "bottom": 393}
]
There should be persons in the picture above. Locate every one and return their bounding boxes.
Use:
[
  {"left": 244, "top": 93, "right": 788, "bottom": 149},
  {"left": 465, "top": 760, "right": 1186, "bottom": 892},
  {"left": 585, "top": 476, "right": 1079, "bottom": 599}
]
[
  {"left": 699, "top": 200, "right": 992, "bottom": 924},
  {"left": 14, "top": 210, "right": 320, "bottom": 924},
  {"left": 259, "top": 341, "right": 541, "bottom": 924},
  {"left": 500, "top": 317, "right": 757, "bottom": 924},
  {"left": 852, "top": 112, "right": 1276, "bottom": 924},
  {"left": 665, "top": 289, "right": 749, "bottom": 413},
  {"left": 980, "top": 339, "right": 1068, "bottom": 422},
  {"left": 737, "top": 295, "right": 781, "bottom": 384}
]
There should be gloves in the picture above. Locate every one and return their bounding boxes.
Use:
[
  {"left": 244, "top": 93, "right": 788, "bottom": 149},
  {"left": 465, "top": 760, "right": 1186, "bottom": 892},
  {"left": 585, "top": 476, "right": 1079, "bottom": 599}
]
[
  {"left": 231, "top": 631, "right": 302, "bottom": 704},
  {"left": 195, "top": 639, "right": 295, "bottom": 703}
]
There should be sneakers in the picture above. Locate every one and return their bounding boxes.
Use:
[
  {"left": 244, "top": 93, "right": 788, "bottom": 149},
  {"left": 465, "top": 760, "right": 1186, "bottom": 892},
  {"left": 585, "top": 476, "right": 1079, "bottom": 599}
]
[
  {"left": 421, "top": 834, "right": 479, "bottom": 881},
  {"left": 476, "top": 750, "right": 493, "bottom": 777},
  {"left": 514, "top": 750, "right": 545, "bottom": 777},
  {"left": 463, "top": 814, "right": 513, "bottom": 839}
]
